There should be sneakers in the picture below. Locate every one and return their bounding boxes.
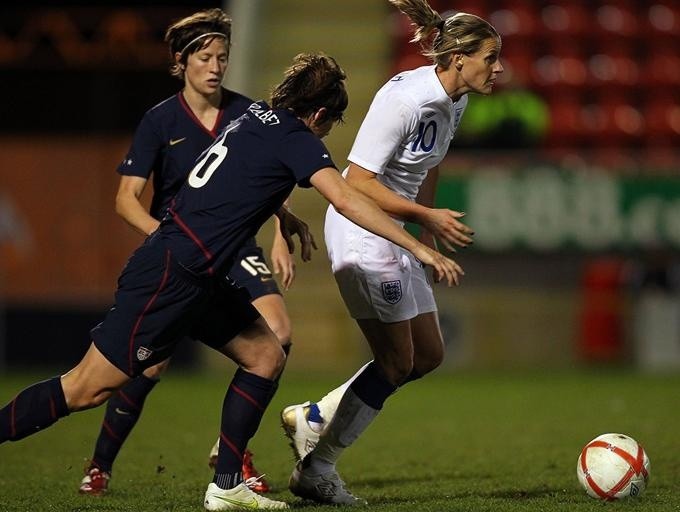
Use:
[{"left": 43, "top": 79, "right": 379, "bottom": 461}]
[
  {"left": 204, "top": 480, "right": 290, "bottom": 511},
  {"left": 207, "top": 437, "right": 274, "bottom": 492},
  {"left": 278, "top": 399, "right": 323, "bottom": 460},
  {"left": 285, "top": 459, "right": 369, "bottom": 507},
  {"left": 76, "top": 466, "right": 113, "bottom": 499}
]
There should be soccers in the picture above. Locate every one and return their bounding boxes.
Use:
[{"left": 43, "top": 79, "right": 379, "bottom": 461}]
[{"left": 577, "top": 433, "right": 650, "bottom": 501}]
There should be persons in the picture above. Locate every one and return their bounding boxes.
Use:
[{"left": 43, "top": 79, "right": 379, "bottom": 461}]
[
  {"left": 87, "top": 9, "right": 164, "bottom": 142},
  {"left": 0, "top": 47, "right": 470, "bottom": 509},
  {"left": 276, "top": 1, "right": 505, "bottom": 506},
  {"left": 1, "top": 13, "right": 87, "bottom": 136},
  {"left": 449, "top": 63, "right": 550, "bottom": 149},
  {"left": 73, "top": 6, "right": 298, "bottom": 497}
]
